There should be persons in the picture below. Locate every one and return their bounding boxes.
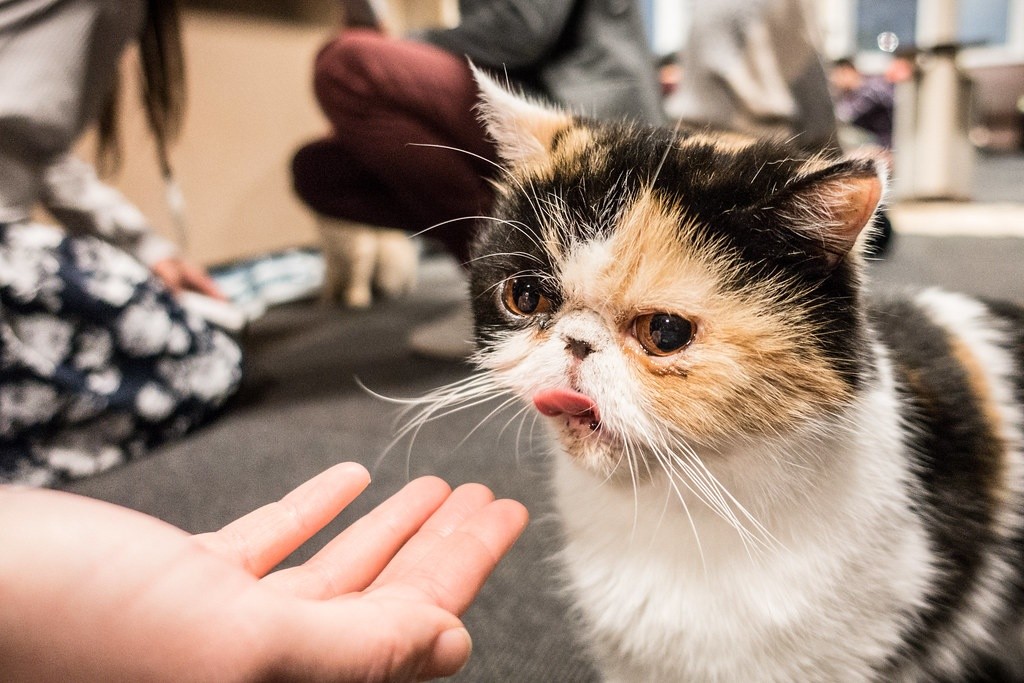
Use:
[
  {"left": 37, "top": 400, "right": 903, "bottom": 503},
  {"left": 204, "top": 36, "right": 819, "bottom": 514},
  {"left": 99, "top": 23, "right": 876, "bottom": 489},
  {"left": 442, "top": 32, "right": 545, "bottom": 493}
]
[
  {"left": 827, "top": 62, "right": 894, "bottom": 148},
  {"left": 293, "top": 0, "right": 659, "bottom": 268},
  {"left": 0, "top": 460, "right": 529, "bottom": 683},
  {"left": 0, "top": 0, "right": 246, "bottom": 489}
]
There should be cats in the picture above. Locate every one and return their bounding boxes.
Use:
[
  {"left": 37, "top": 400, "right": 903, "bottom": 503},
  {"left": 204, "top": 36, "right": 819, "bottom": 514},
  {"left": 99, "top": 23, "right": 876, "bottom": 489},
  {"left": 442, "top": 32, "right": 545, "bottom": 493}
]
[
  {"left": 318, "top": 216, "right": 417, "bottom": 309},
  {"left": 352, "top": 52, "right": 1024, "bottom": 683}
]
